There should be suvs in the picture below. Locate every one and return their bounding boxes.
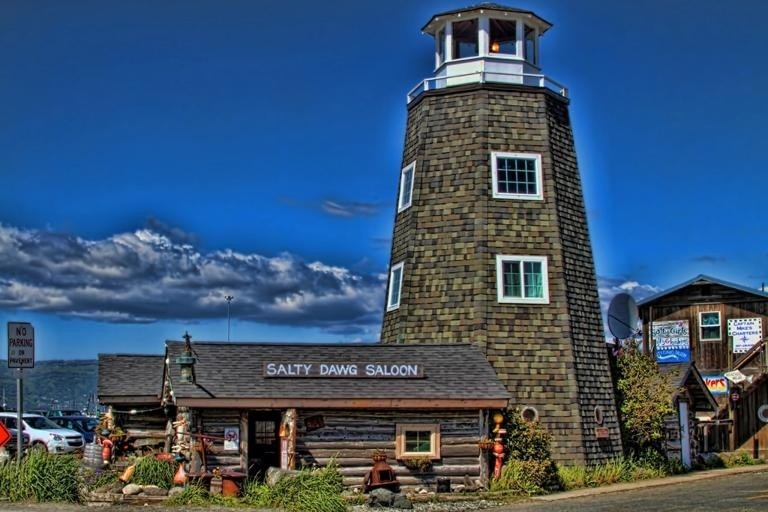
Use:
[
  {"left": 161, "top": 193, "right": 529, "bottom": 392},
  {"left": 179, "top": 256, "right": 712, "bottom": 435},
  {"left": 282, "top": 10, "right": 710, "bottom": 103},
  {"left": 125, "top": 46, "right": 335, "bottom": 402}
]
[{"left": 0, "top": 411, "right": 86, "bottom": 455}]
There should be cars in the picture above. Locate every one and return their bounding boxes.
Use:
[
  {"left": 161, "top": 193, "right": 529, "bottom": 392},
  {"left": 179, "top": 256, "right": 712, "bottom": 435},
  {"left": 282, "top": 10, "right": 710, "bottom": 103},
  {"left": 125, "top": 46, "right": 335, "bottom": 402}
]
[{"left": 46, "top": 408, "right": 99, "bottom": 441}]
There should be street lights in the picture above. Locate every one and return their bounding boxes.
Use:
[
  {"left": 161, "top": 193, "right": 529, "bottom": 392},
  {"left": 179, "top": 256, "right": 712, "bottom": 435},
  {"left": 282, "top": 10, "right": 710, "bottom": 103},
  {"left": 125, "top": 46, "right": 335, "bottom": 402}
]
[{"left": 225, "top": 295, "right": 233, "bottom": 341}]
